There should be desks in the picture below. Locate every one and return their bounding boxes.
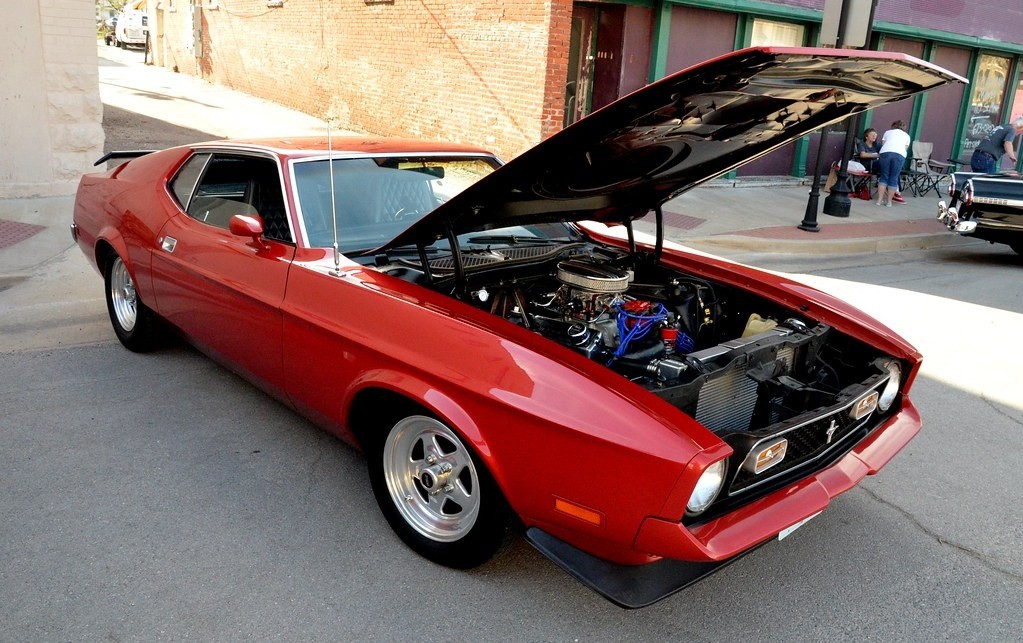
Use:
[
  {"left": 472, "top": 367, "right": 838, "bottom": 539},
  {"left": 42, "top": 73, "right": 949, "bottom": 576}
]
[
  {"left": 833, "top": 165, "right": 872, "bottom": 200},
  {"left": 947, "top": 158, "right": 971, "bottom": 172}
]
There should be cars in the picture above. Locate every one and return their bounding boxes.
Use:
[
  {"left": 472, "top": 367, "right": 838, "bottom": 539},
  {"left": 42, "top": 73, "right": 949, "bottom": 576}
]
[
  {"left": 936, "top": 172, "right": 1023, "bottom": 256},
  {"left": 114, "top": 11, "right": 148, "bottom": 49},
  {"left": 70, "top": 46, "right": 969, "bottom": 611}
]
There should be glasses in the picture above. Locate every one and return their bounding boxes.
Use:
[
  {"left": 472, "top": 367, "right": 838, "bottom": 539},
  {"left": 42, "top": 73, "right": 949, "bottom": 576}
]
[{"left": 868, "top": 133, "right": 877, "bottom": 136}]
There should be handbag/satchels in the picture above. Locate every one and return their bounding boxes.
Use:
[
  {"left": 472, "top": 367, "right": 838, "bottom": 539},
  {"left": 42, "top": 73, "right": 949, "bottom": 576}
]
[{"left": 857, "top": 184, "right": 869, "bottom": 200}]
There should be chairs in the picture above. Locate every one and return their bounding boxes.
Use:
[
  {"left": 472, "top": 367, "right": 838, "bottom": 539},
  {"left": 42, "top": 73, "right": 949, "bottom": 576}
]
[
  {"left": 855, "top": 137, "right": 955, "bottom": 198},
  {"left": 368, "top": 170, "right": 435, "bottom": 224},
  {"left": 244, "top": 172, "right": 328, "bottom": 243}
]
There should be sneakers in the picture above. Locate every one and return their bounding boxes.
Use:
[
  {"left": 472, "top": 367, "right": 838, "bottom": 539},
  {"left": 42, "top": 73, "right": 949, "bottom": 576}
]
[{"left": 893, "top": 193, "right": 906, "bottom": 204}]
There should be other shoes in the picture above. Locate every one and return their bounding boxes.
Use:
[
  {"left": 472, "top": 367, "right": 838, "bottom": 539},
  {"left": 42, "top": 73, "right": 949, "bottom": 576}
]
[
  {"left": 886, "top": 202, "right": 891, "bottom": 207},
  {"left": 876, "top": 199, "right": 883, "bottom": 205}
]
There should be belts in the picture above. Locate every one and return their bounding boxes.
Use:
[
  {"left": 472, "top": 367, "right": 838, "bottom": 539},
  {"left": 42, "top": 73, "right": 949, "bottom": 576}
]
[{"left": 975, "top": 149, "right": 996, "bottom": 161}]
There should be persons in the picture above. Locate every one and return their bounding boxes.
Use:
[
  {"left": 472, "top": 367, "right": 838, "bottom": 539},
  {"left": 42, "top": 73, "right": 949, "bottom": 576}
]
[
  {"left": 858, "top": 128, "right": 907, "bottom": 204},
  {"left": 875, "top": 120, "right": 911, "bottom": 207},
  {"left": 970, "top": 115, "right": 1023, "bottom": 176}
]
